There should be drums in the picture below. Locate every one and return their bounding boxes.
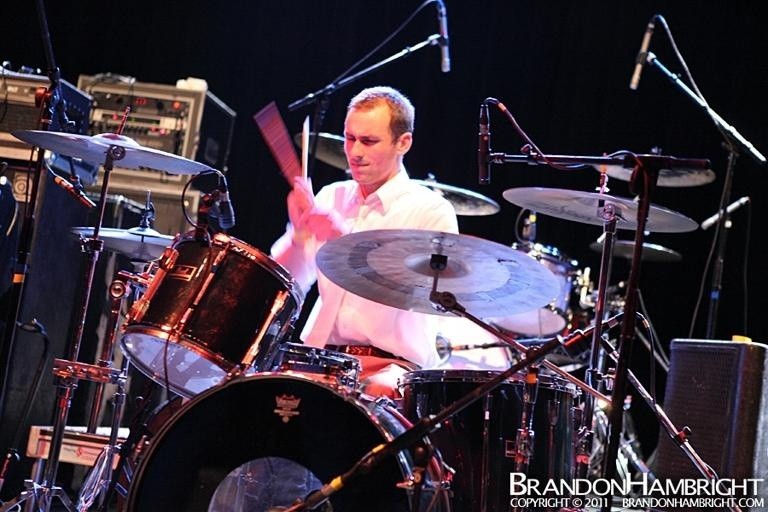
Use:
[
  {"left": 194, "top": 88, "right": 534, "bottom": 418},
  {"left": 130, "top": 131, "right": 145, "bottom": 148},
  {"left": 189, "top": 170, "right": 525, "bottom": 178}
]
[
  {"left": 106, "top": 373, "right": 454, "bottom": 512},
  {"left": 397, "top": 369, "right": 577, "bottom": 512},
  {"left": 118, "top": 228, "right": 304, "bottom": 400},
  {"left": 486, "top": 241, "right": 579, "bottom": 337},
  {"left": 269, "top": 342, "right": 359, "bottom": 400}
]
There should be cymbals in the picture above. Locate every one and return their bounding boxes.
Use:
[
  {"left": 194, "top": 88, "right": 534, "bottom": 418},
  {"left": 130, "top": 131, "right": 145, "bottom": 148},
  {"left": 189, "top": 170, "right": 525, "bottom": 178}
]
[
  {"left": 315, "top": 228, "right": 561, "bottom": 318},
  {"left": 410, "top": 180, "right": 501, "bottom": 217},
  {"left": 11, "top": 128, "right": 216, "bottom": 177},
  {"left": 72, "top": 225, "right": 175, "bottom": 263},
  {"left": 591, "top": 240, "right": 681, "bottom": 265},
  {"left": 591, "top": 152, "right": 716, "bottom": 187},
  {"left": 503, "top": 186, "right": 700, "bottom": 234},
  {"left": 293, "top": 132, "right": 349, "bottom": 170}
]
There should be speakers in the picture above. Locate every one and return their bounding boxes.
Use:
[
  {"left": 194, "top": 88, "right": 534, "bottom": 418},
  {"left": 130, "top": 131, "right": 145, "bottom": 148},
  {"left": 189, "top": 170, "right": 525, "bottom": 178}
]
[{"left": 654, "top": 336, "right": 768, "bottom": 511}]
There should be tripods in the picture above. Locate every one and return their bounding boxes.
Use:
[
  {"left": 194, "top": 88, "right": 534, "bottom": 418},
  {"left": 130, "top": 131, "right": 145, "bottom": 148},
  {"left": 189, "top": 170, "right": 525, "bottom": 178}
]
[{"left": 0, "top": 158, "right": 113, "bottom": 512}]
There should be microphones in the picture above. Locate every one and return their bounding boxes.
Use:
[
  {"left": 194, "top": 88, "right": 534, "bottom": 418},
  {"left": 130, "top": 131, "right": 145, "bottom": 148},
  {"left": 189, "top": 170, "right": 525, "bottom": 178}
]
[
  {"left": 478, "top": 105, "right": 491, "bottom": 185},
  {"left": 562, "top": 310, "right": 632, "bottom": 360},
  {"left": 55, "top": 176, "right": 97, "bottom": 208},
  {"left": 701, "top": 197, "right": 746, "bottom": 231},
  {"left": 218, "top": 175, "right": 236, "bottom": 228},
  {"left": 439, "top": 5, "right": 451, "bottom": 72},
  {"left": 629, "top": 22, "right": 655, "bottom": 90}
]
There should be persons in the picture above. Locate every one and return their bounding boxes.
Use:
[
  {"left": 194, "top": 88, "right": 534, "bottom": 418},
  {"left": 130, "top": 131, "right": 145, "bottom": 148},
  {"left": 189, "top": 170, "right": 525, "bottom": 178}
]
[{"left": 268, "top": 85, "right": 459, "bottom": 400}]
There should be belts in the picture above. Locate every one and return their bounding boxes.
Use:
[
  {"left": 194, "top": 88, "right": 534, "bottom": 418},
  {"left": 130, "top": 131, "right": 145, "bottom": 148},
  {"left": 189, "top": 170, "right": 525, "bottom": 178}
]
[{"left": 325, "top": 344, "right": 402, "bottom": 360}]
